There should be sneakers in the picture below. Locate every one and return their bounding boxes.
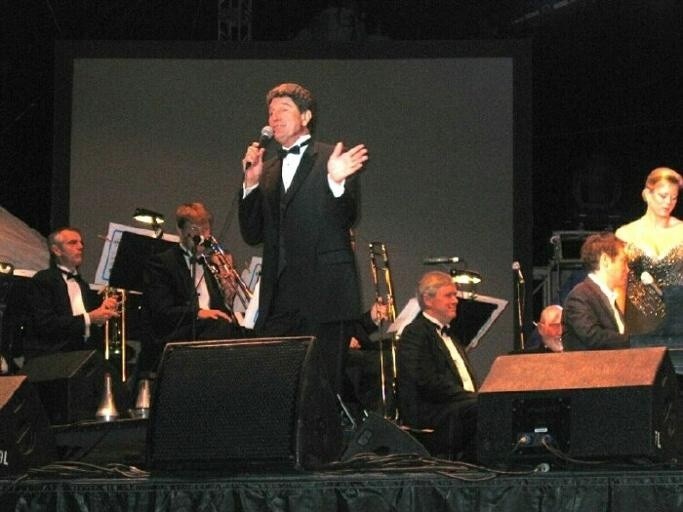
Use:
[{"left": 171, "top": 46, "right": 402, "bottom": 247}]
[{"left": 278, "top": 146, "right": 301, "bottom": 158}]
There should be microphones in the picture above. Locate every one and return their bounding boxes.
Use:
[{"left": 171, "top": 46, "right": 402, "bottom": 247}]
[
  {"left": 192, "top": 231, "right": 211, "bottom": 248},
  {"left": 421, "top": 256, "right": 462, "bottom": 265},
  {"left": 512, "top": 261, "right": 526, "bottom": 287},
  {"left": 640, "top": 271, "right": 664, "bottom": 299},
  {"left": 244, "top": 125, "right": 274, "bottom": 180}
]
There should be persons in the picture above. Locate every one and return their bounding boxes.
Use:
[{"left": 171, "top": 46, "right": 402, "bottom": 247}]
[
  {"left": 391, "top": 268, "right": 483, "bottom": 458},
  {"left": 342, "top": 292, "right": 397, "bottom": 403},
  {"left": 505, "top": 302, "right": 565, "bottom": 353},
  {"left": 560, "top": 230, "right": 631, "bottom": 350},
  {"left": 237, "top": 81, "right": 371, "bottom": 462},
  {"left": 135, "top": 200, "right": 263, "bottom": 344},
  {"left": 611, "top": 167, "right": 683, "bottom": 345},
  {"left": 19, "top": 226, "right": 126, "bottom": 423}
]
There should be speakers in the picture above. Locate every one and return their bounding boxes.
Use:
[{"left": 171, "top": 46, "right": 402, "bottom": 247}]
[
  {"left": 474, "top": 346, "right": 682, "bottom": 461},
  {"left": 0, "top": 373, "right": 62, "bottom": 478},
  {"left": 145, "top": 336, "right": 343, "bottom": 473}
]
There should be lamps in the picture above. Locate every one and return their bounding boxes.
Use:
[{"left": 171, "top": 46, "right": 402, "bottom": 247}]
[
  {"left": 450, "top": 267, "right": 482, "bottom": 298},
  {"left": 132, "top": 207, "right": 166, "bottom": 238}
]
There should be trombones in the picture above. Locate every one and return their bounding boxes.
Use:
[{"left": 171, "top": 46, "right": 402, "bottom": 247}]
[
  {"left": 368, "top": 241, "right": 399, "bottom": 426},
  {"left": 93, "top": 287, "right": 125, "bottom": 423},
  {"left": 200, "top": 236, "right": 253, "bottom": 328}
]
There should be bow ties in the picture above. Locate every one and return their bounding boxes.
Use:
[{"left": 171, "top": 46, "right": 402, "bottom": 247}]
[
  {"left": 440, "top": 326, "right": 452, "bottom": 335},
  {"left": 67, "top": 272, "right": 81, "bottom": 281},
  {"left": 189, "top": 257, "right": 205, "bottom": 266}
]
